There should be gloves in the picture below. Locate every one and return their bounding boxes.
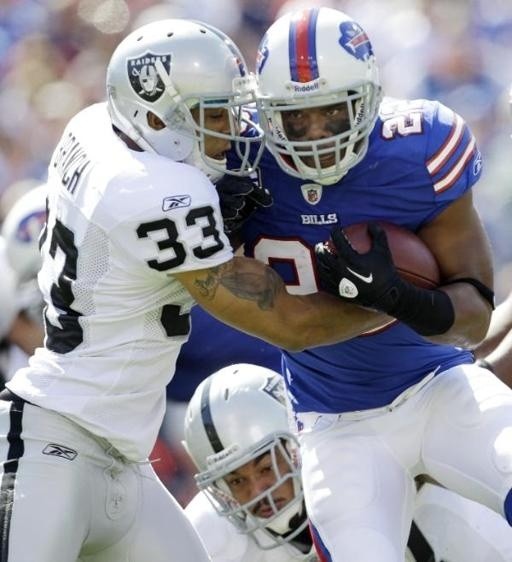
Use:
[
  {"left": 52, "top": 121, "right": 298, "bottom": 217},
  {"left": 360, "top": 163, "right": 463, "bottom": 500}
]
[
  {"left": 215, "top": 168, "right": 275, "bottom": 235},
  {"left": 314, "top": 219, "right": 406, "bottom": 314}
]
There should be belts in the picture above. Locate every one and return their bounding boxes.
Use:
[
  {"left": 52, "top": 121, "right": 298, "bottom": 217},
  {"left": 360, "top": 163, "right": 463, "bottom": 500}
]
[{"left": 96, "top": 437, "right": 128, "bottom": 460}]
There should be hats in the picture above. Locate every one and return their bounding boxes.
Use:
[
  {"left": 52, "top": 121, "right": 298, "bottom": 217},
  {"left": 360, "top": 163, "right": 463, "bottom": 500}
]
[{"left": 254, "top": 4, "right": 383, "bottom": 187}]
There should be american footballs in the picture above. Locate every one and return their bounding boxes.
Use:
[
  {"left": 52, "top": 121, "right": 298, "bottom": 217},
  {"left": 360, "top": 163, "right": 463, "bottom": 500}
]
[{"left": 315, "top": 221, "right": 441, "bottom": 292}]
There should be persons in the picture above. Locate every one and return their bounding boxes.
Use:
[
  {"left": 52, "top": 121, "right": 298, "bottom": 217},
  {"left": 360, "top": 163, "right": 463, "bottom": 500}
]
[
  {"left": 212, "top": 4, "right": 512, "bottom": 561},
  {"left": 1, "top": 185, "right": 46, "bottom": 384},
  {"left": 1, "top": 21, "right": 396, "bottom": 562},
  {"left": 185, "top": 363, "right": 511, "bottom": 562},
  {"left": 0, "top": 0, "right": 511, "bottom": 501}
]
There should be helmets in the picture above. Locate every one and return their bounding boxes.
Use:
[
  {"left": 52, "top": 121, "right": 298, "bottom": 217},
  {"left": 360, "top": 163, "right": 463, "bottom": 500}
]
[
  {"left": 106, "top": 18, "right": 266, "bottom": 186},
  {"left": 182, "top": 363, "right": 309, "bottom": 550}
]
